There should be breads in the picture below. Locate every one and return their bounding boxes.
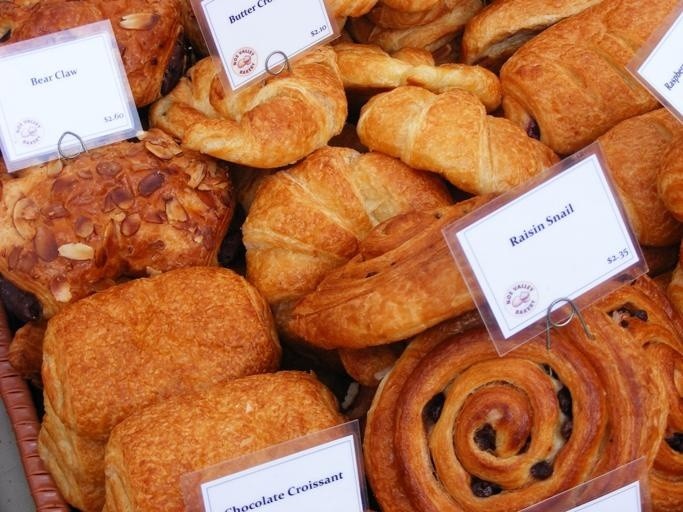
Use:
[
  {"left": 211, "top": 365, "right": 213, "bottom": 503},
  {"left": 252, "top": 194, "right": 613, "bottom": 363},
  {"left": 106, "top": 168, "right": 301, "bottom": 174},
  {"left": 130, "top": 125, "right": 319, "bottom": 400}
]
[{"left": 0, "top": 0, "right": 683, "bottom": 512}]
